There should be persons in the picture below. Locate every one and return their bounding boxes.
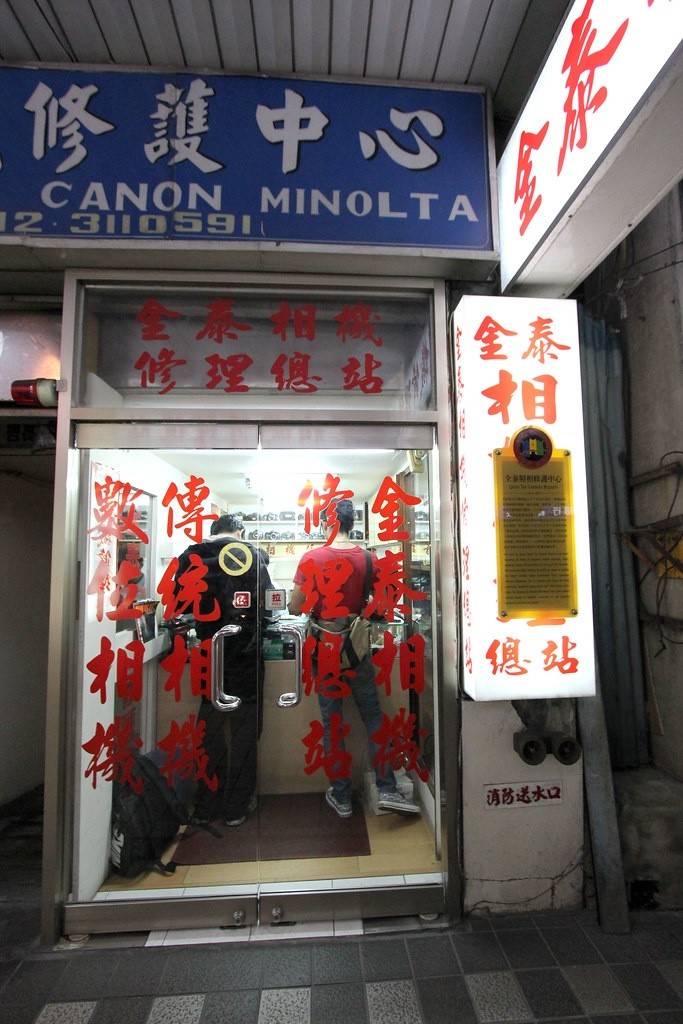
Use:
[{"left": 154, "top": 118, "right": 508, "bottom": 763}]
[
  {"left": 173, "top": 514, "right": 276, "bottom": 826},
  {"left": 120, "top": 558, "right": 145, "bottom": 602},
  {"left": 287, "top": 499, "right": 420, "bottom": 819}
]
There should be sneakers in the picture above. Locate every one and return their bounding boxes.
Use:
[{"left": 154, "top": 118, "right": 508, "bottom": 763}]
[
  {"left": 377, "top": 790, "right": 421, "bottom": 817},
  {"left": 225, "top": 795, "right": 258, "bottom": 826},
  {"left": 325, "top": 787, "right": 353, "bottom": 818}
]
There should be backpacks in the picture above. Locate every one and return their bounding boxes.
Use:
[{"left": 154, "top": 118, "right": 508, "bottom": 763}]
[{"left": 110, "top": 748, "right": 187, "bottom": 880}]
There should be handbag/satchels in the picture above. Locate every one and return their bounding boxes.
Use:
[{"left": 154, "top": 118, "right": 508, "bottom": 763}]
[{"left": 303, "top": 549, "right": 375, "bottom": 675}]
